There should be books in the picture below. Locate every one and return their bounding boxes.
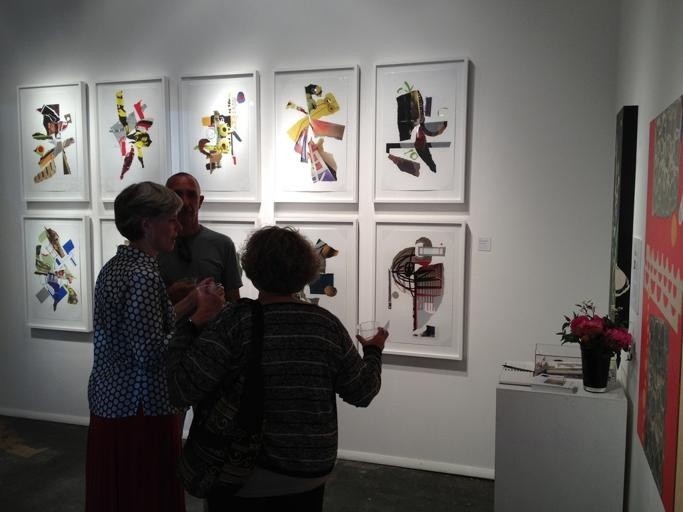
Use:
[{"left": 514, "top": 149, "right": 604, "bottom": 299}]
[{"left": 529, "top": 376, "right": 578, "bottom": 395}]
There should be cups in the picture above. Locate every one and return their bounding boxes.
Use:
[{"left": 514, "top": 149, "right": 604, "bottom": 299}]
[
  {"left": 177, "top": 277, "right": 196, "bottom": 287},
  {"left": 355, "top": 322, "right": 381, "bottom": 340},
  {"left": 196, "top": 284, "right": 221, "bottom": 301}
]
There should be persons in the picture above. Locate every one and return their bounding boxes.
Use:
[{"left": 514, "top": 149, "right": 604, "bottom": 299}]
[
  {"left": 170, "top": 225, "right": 389, "bottom": 511},
  {"left": 148, "top": 171, "right": 242, "bottom": 420},
  {"left": 82, "top": 180, "right": 189, "bottom": 512}
]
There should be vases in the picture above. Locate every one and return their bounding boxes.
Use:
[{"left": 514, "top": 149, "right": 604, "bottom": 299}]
[{"left": 579, "top": 344, "right": 610, "bottom": 394}]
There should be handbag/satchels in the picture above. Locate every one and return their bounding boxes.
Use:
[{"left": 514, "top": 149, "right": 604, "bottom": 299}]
[{"left": 183, "top": 426, "right": 259, "bottom": 497}]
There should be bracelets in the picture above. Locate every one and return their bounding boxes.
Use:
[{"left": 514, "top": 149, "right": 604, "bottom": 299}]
[{"left": 185, "top": 318, "right": 196, "bottom": 328}]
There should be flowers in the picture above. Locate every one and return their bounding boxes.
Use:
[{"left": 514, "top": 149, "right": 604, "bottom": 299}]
[{"left": 559, "top": 302, "right": 633, "bottom": 369}]
